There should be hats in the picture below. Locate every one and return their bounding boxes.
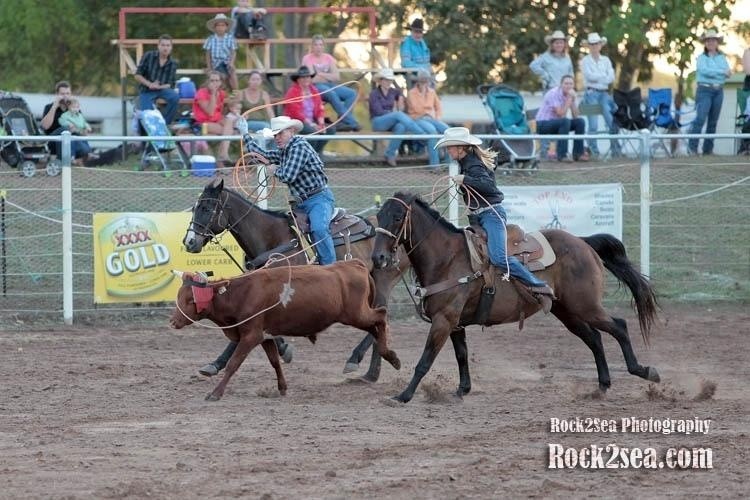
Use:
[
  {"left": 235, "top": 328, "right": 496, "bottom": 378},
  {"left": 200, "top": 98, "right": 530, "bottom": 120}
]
[
  {"left": 408, "top": 69, "right": 434, "bottom": 81},
  {"left": 582, "top": 32, "right": 608, "bottom": 47},
  {"left": 404, "top": 19, "right": 426, "bottom": 31},
  {"left": 373, "top": 69, "right": 395, "bottom": 84},
  {"left": 698, "top": 30, "right": 723, "bottom": 44},
  {"left": 434, "top": 127, "right": 483, "bottom": 149},
  {"left": 263, "top": 116, "right": 304, "bottom": 138},
  {"left": 545, "top": 30, "right": 567, "bottom": 46},
  {"left": 290, "top": 66, "right": 317, "bottom": 83},
  {"left": 206, "top": 14, "right": 236, "bottom": 33}
]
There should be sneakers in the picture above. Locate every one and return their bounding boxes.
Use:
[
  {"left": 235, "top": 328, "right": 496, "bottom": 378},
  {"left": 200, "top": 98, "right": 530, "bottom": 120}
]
[
  {"left": 383, "top": 154, "right": 397, "bottom": 167},
  {"left": 72, "top": 152, "right": 100, "bottom": 166},
  {"left": 336, "top": 122, "right": 364, "bottom": 132},
  {"left": 557, "top": 155, "right": 590, "bottom": 162},
  {"left": 539, "top": 284, "right": 552, "bottom": 314}
]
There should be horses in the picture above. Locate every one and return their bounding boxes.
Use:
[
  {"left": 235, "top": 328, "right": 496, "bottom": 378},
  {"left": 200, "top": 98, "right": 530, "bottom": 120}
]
[
  {"left": 371, "top": 190, "right": 662, "bottom": 405},
  {"left": 181, "top": 180, "right": 411, "bottom": 385}
]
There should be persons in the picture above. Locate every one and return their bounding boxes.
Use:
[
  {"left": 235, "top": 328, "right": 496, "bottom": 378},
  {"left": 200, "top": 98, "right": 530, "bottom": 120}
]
[
  {"left": 40, "top": 14, "right": 750, "bottom": 168},
  {"left": 432, "top": 126, "right": 553, "bottom": 317},
  {"left": 234, "top": 113, "right": 336, "bottom": 267}
]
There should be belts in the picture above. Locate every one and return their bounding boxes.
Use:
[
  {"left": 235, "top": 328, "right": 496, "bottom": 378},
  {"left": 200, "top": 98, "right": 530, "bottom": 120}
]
[
  {"left": 698, "top": 82, "right": 723, "bottom": 89},
  {"left": 299, "top": 184, "right": 326, "bottom": 200},
  {"left": 472, "top": 203, "right": 502, "bottom": 215},
  {"left": 588, "top": 86, "right": 609, "bottom": 93}
]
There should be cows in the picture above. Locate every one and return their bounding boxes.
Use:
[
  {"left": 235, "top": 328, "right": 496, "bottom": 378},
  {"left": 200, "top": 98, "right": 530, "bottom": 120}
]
[{"left": 166, "top": 257, "right": 402, "bottom": 403}]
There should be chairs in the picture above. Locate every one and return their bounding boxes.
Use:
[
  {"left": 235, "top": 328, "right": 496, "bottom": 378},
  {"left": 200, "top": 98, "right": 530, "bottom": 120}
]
[
  {"left": 613, "top": 87, "right": 672, "bottom": 161},
  {"left": 733, "top": 89, "right": 750, "bottom": 158},
  {"left": 526, "top": 107, "right": 571, "bottom": 162},
  {"left": 646, "top": 88, "right": 702, "bottom": 161},
  {"left": 579, "top": 104, "right": 639, "bottom": 162}
]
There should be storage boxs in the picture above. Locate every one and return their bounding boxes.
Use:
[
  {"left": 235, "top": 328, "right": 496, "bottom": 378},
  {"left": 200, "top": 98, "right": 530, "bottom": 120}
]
[{"left": 190, "top": 155, "right": 217, "bottom": 177}]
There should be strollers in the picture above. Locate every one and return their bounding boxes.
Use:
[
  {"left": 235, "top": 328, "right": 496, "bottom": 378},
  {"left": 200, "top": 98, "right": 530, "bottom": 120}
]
[
  {"left": 0, "top": 92, "right": 61, "bottom": 180},
  {"left": 129, "top": 99, "right": 189, "bottom": 178},
  {"left": 475, "top": 81, "right": 541, "bottom": 179}
]
